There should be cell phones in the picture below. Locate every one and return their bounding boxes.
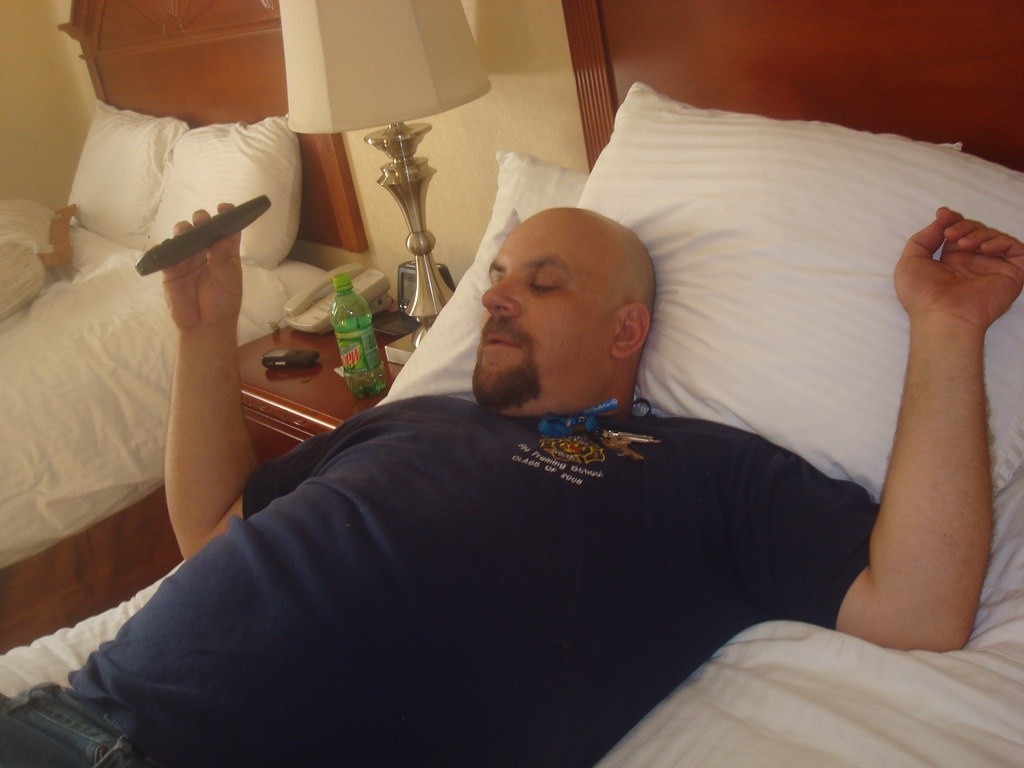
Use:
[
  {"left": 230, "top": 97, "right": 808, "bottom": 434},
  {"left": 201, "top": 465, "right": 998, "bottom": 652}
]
[{"left": 261, "top": 347, "right": 320, "bottom": 369}]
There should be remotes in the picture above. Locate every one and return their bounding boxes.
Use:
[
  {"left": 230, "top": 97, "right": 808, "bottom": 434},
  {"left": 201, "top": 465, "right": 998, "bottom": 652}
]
[{"left": 135, "top": 196, "right": 272, "bottom": 278}]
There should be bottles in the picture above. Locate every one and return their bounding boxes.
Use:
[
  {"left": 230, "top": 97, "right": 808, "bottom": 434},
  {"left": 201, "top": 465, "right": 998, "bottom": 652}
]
[{"left": 329, "top": 273, "right": 387, "bottom": 399}]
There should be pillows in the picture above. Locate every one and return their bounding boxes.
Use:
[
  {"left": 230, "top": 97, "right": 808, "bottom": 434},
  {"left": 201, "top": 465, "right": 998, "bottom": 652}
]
[
  {"left": 373, "top": 152, "right": 589, "bottom": 411},
  {"left": 68, "top": 98, "right": 190, "bottom": 250},
  {"left": 575, "top": 80, "right": 1024, "bottom": 642},
  {"left": 147, "top": 115, "right": 303, "bottom": 270}
]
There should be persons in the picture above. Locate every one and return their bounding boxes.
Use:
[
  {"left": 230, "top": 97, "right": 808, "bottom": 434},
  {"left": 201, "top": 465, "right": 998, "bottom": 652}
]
[{"left": 0, "top": 204, "right": 1024, "bottom": 768}]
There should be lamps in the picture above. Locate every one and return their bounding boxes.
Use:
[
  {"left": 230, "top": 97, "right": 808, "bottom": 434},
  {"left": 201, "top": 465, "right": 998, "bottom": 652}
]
[{"left": 275, "top": 0, "right": 490, "bottom": 365}]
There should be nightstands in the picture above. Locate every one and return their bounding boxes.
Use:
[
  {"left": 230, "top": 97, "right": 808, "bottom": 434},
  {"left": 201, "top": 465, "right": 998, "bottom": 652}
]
[{"left": 235, "top": 330, "right": 398, "bottom": 462}]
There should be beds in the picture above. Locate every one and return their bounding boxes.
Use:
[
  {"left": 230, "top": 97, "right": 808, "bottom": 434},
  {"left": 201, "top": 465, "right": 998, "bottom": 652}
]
[
  {"left": 0, "top": 0, "right": 1024, "bottom": 768},
  {"left": 0, "top": 0, "right": 368, "bottom": 652}
]
